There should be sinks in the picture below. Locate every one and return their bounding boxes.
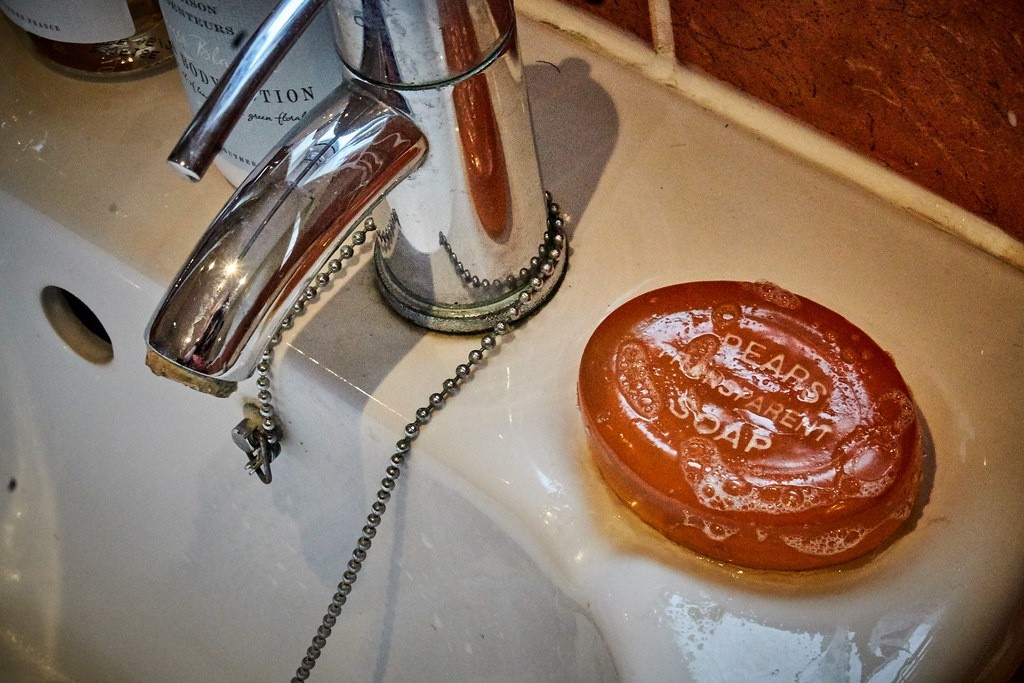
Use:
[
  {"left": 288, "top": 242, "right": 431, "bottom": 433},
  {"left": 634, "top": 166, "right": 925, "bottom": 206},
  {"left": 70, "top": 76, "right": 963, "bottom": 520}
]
[{"left": 0, "top": 1, "right": 1022, "bottom": 683}]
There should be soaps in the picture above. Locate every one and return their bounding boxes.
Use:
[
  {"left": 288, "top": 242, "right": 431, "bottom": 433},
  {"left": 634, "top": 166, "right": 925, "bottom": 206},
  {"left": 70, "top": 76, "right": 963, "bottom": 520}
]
[{"left": 576, "top": 280, "right": 935, "bottom": 573}]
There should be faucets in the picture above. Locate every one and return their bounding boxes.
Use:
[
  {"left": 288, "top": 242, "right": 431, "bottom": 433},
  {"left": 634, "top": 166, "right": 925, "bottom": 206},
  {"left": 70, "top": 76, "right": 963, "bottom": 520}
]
[{"left": 146, "top": 5, "right": 569, "bottom": 399}]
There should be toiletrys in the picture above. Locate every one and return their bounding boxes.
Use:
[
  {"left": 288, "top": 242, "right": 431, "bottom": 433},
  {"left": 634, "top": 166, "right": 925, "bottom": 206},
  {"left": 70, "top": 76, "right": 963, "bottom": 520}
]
[
  {"left": 155, "top": 0, "right": 348, "bottom": 193},
  {"left": 3, "top": 1, "right": 169, "bottom": 81}
]
